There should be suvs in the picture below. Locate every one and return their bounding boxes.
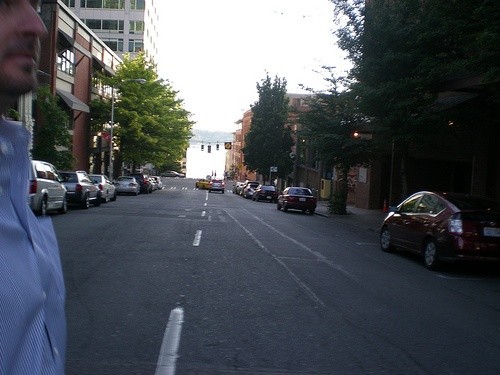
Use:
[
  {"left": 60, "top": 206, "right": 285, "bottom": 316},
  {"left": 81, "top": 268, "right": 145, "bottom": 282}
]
[
  {"left": 57, "top": 170, "right": 102, "bottom": 209},
  {"left": 28, "top": 159, "right": 68, "bottom": 217}
]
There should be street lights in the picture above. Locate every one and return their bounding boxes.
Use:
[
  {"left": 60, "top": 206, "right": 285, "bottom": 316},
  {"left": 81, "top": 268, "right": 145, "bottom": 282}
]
[{"left": 109, "top": 79, "right": 146, "bottom": 182}]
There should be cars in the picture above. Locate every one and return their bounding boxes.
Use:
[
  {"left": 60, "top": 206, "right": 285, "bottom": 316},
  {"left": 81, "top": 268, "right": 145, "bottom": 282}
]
[
  {"left": 115, "top": 173, "right": 162, "bottom": 196},
  {"left": 379, "top": 191, "right": 500, "bottom": 271},
  {"left": 233, "top": 179, "right": 279, "bottom": 203},
  {"left": 277, "top": 187, "right": 317, "bottom": 216},
  {"left": 195, "top": 179, "right": 225, "bottom": 194},
  {"left": 87, "top": 174, "right": 116, "bottom": 204},
  {"left": 161, "top": 171, "right": 185, "bottom": 178}
]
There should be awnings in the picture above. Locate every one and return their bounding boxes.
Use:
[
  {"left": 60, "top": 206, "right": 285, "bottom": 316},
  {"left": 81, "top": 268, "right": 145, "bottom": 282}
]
[
  {"left": 56, "top": 88, "right": 90, "bottom": 122},
  {"left": 57, "top": 28, "right": 91, "bottom": 68}
]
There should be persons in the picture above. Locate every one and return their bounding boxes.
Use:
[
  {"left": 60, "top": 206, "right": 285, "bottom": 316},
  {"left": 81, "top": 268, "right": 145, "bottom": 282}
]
[{"left": 0, "top": 1, "right": 69, "bottom": 375}]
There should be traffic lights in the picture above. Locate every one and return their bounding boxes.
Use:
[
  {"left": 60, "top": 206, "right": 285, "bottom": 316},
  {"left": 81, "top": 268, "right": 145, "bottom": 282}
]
[
  {"left": 217, "top": 144, "right": 219, "bottom": 150},
  {"left": 201, "top": 145, "right": 204, "bottom": 151}
]
